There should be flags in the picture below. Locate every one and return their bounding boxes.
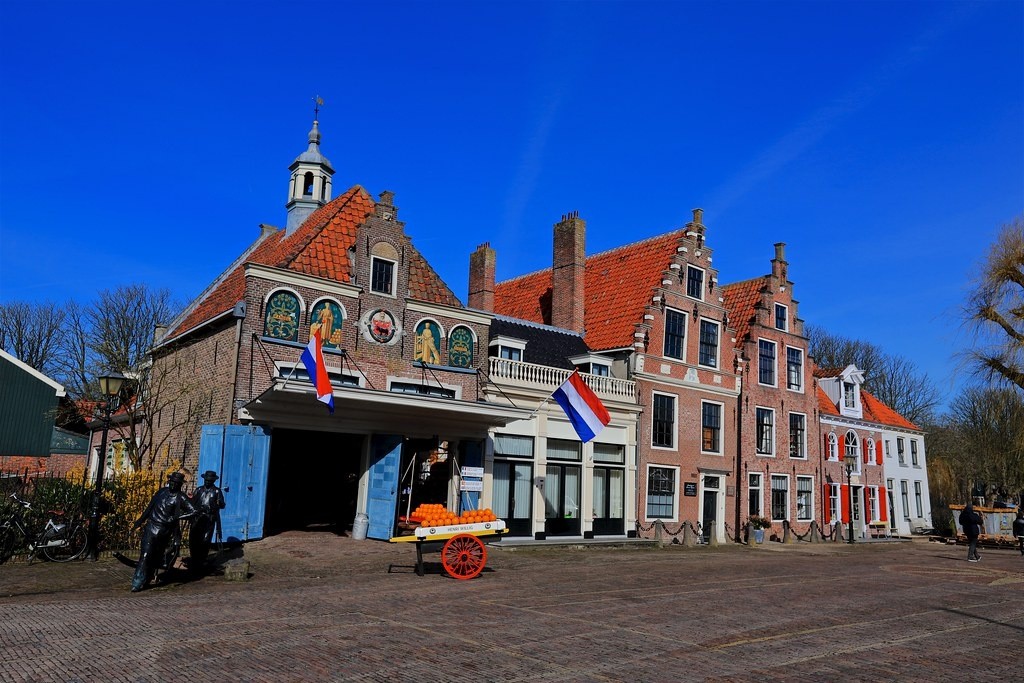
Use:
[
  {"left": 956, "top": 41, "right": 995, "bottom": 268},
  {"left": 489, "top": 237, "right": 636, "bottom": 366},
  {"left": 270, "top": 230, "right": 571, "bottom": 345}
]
[
  {"left": 300, "top": 328, "right": 339, "bottom": 416},
  {"left": 551, "top": 372, "right": 612, "bottom": 444}
]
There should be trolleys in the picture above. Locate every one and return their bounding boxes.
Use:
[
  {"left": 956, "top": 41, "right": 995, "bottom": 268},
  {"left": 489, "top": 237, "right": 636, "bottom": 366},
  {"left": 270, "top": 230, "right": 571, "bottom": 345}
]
[{"left": 388, "top": 520, "right": 509, "bottom": 581}]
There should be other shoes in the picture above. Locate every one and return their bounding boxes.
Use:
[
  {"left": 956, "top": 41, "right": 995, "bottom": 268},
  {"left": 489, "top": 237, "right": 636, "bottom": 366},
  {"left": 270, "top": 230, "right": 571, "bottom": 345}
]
[
  {"left": 968, "top": 558, "right": 978, "bottom": 563},
  {"left": 976, "top": 556, "right": 982, "bottom": 561}
]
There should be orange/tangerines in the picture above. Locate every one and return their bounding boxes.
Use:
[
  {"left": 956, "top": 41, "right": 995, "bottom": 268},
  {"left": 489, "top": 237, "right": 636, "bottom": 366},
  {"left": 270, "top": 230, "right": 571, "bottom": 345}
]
[{"left": 412, "top": 504, "right": 496, "bottom": 527}]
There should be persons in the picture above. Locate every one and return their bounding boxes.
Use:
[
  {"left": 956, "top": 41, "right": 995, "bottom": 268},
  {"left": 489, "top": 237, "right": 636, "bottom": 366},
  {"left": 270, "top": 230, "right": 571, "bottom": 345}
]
[
  {"left": 188, "top": 471, "right": 226, "bottom": 578},
  {"left": 130, "top": 472, "right": 198, "bottom": 592},
  {"left": 1013, "top": 509, "right": 1024, "bottom": 556},
  {"left": 959, "top": 504, "right": 983, "bottom": 562}
]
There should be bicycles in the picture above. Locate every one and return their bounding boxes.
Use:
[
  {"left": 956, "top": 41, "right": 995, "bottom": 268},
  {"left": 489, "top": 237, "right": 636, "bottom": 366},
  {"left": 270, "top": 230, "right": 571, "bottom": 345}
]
[{"left": 0, "top": 492, "right": 89, "bottom": 567}]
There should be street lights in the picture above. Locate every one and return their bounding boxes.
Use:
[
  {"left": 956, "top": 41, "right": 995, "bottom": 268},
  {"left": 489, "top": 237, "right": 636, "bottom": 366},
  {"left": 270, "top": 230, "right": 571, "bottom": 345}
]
[
  {"left": 90, "top": 370, "right": 127, "bottom": 561},
  {"left": 842, "top": 451, "right": 859, "bottom": 543}
]
